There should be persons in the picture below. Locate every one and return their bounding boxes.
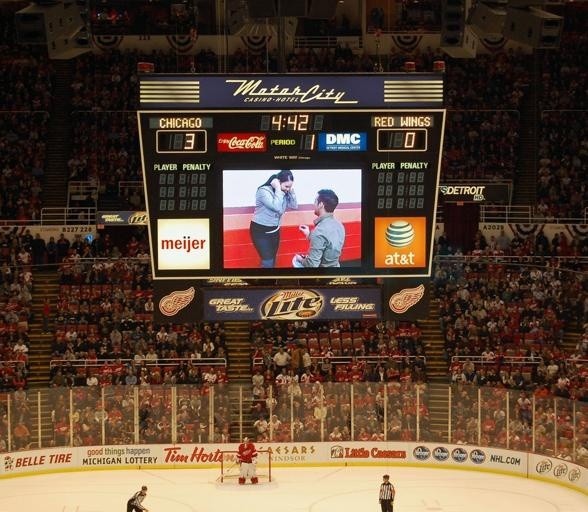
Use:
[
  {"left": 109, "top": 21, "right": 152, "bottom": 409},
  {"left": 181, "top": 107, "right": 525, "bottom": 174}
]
[
  {"left": 432, "top": 231, "right": 587, "bottom": 465},
  {"left": 126, "top": 485, "right": 149, "bottom": 512},
  {"left": 378, "top": 474, "right": 395, "bottom": 512},
  {"left": 248, "top": 169, "right": 298, "bottom": 268},
  {"left": 236, "top": 436, "right": 258, "bottom": 484},
  {"left": 292, "top": 188, "right": 345, "bottom": 267},
  {"left": 47, "top": 233, "right": 227, "bottom": 446},
  {"left": 249, "top": 320, "right": 431, "bottom": 442},
  {"left": 2, "top": 1, "right": 586, "bottom": 224},
  {"left": 2, "top": 229, "right": 46, "bottom": 452}
]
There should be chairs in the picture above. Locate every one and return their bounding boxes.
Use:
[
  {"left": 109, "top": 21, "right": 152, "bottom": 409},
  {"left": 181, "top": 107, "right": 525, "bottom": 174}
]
[
  {"left": 439, "top": 257, "right": 588, "bottom": 465},
  {"left": 0, "top": 257, "right": 33, "bottom": 452},
  {"left": 250, "top": 318, "right": 428, "bottom": 443},
  {"left": 51, "top": 256, "right": 230, "bottom": 445}
]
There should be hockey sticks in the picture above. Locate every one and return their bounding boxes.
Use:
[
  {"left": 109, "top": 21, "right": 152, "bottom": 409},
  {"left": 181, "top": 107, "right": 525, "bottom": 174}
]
[{"left": 207, "top": 459, "right": 237, "bottom": 483}]
